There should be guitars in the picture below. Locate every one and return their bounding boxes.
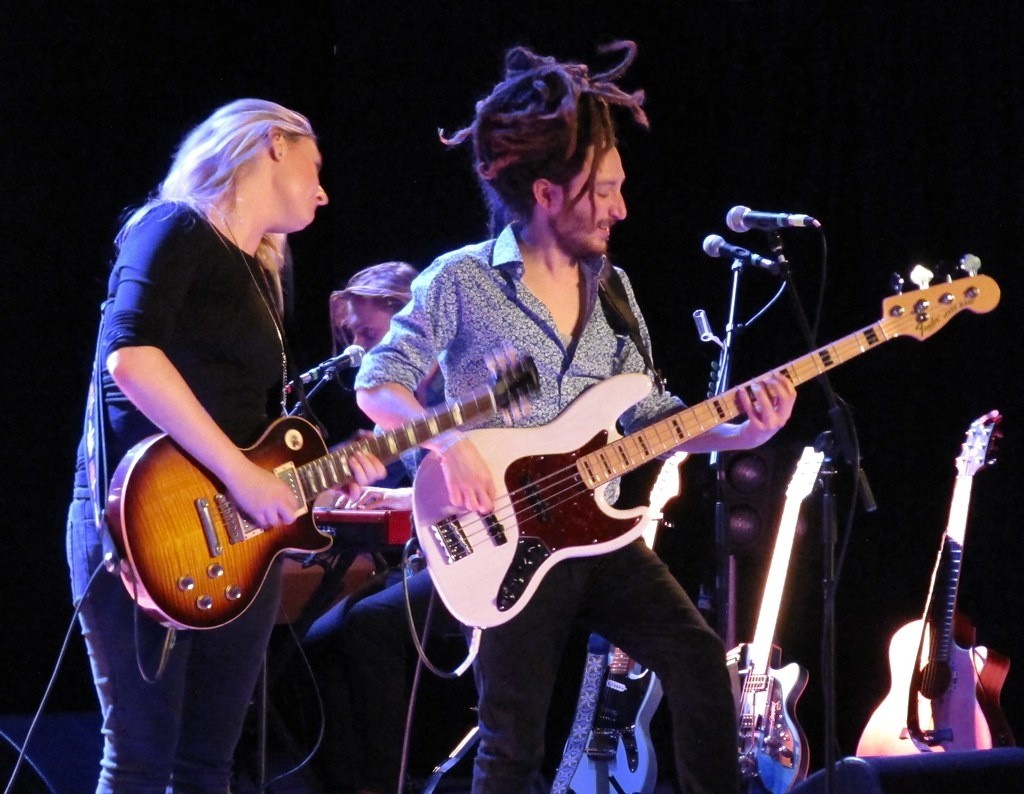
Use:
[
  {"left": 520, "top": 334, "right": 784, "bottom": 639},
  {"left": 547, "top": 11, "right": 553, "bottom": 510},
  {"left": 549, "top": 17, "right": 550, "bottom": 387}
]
[
  {"left": 411, "top": 253, "right": 1002, "bottom": 628},
  {"left": 106, "top": 344, "right": 542, "bottom": 632},
  {"left": 562, "top": 454, "right": 683, "bottom": 794},
  {"left": 725, "top": 444, "right": 822, "bottom": 794},
  {"left": 855, "top": 406, "right": 1016, "bottom": 760}
]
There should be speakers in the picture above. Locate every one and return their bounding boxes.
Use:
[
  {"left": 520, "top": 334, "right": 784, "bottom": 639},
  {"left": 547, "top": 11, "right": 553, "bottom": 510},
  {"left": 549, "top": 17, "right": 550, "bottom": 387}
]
[{"left": 784, "top": 747, "right": 1024, "bottom": 794}]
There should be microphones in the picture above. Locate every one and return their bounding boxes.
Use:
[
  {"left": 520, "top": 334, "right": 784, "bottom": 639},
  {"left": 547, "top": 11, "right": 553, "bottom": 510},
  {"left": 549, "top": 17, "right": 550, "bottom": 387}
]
[
  {"left": 702, "top": 233, "right": 777, "bottom": 273},
  {"left": 285, "top": 345, "right": 365, "bottom": 394},
  {"left": 726, "top": 205, "right": 810, "bottom": 233}
]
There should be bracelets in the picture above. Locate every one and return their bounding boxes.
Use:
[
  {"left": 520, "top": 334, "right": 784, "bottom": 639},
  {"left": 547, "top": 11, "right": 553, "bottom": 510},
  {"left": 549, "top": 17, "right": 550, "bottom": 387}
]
[{"left": 437, "top": 435, "right": 468, "bottom": 456}]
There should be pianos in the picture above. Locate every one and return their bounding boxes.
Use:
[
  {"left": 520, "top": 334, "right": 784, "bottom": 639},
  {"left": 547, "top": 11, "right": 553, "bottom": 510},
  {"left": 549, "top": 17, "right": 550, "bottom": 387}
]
[{"left": 251, "top": 508, "right": 415, "bottom": 712}]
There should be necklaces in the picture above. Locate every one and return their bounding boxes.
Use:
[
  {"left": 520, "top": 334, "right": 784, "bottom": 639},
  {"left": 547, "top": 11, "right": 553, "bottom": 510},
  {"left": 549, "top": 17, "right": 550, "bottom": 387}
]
[{"left": 205, "top": 199, "right": 289, "bottom": 415}]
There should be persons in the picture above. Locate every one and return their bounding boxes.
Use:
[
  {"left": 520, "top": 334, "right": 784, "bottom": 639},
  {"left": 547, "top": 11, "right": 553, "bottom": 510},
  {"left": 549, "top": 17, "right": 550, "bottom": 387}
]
[
  {"left": 65, "top": 100, "right": 390, "bottom": 794},
  {"left": 352, "top": 36, "right": 798, "bottom": 794},
  {"left": 323, "top": 264, "right": 463, "bottom": 794}
]
